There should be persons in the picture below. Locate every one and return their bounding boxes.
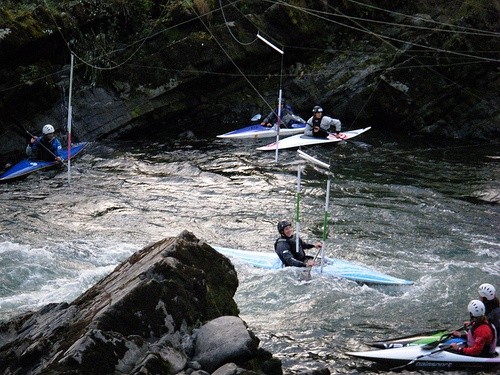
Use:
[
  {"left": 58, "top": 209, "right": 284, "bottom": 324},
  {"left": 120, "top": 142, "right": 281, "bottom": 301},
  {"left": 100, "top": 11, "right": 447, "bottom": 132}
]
[
  {"left": 450, "top": 299, "right": 497, "bottom": 357},
  {"left": 261, "top": 98, "right": 294, "bottom": 129},
  {"left": 304, "top": 106, "right": 341, "bottom": 139},
  {"left": 477, "top": 282, "right": 500, "bottom": 347},
  {"left": 26, "top": 124, "right": 63, "bottom": 162},
  {"left": 274, "top": 221, "right": 322, "bottom": 267}
]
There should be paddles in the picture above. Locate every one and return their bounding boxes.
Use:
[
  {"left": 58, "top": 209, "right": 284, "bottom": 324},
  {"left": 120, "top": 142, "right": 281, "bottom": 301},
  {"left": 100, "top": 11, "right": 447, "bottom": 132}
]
[
  {"left": 388, "top": 341, "right": 466, "bottom": 371},
  {"left": 301, "top": 249, "right": 322, "bottom": 280},
  {"left": 22, "top": 125, "right": 68, "bottom": 169},
  {"left": 252, "top": 113, "right": 263, "bottom": 124},
  {"left": 419, "top": 323, "right": 470, "bottom": 349},
  {"left": 305, "top": 121, "right": 360, "bottom": 146}
]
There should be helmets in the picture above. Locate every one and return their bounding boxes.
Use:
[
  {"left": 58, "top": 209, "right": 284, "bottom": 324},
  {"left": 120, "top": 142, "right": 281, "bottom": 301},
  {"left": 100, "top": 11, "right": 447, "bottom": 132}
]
[
  {"left": 468, "top": 299, "right": 486, "bottom": 316},
  {"left": 278, "top": 219, "right": 292, "bottom": 234},
  {"left": 276, "top": 98, "right": 286, "bottom": 104},
  {"left": 312, "top": 106, "right": 323, "bottom": 113},
  {"left": 479, "top": 283, "right": 497, "bottom": 300},
  {"left": 42, "top": 123, "right": 55, "bottom": 134}
]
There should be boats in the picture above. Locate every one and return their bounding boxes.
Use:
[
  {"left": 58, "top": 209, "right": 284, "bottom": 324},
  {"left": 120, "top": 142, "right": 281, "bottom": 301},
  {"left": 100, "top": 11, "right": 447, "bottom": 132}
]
[
  {"left": 253, "top": 127, "right": 373, "bottom": 153},
  {"left": 0, "top": 142, "right": 89, "bottom": 181},
  {"left": 365, "top": 327, "right": 459, "bottom": 351},
  {"left": 204, "top": 150, "right": 417, "bottom": 287},
  {"left": 215, "top": 116, "right": 308, "bottom": 141},
  {"left": 345, "top": 339, "right": 500, "bottom": 371}
]
